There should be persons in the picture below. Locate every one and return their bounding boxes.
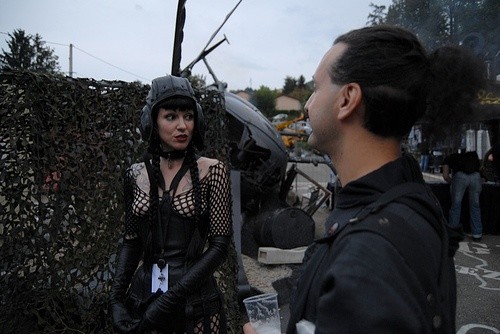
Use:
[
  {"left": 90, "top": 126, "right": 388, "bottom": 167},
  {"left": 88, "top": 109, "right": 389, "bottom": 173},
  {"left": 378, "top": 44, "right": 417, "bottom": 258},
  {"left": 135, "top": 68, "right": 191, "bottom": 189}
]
[
  {"left": 419, "top": 138, "right": 430, "bottom": 172},
  {"left": 243, "top": 26, "right": 485, "bottom": 334},
  {"left": 108, "top": 75, "right": 233, "bottom": 334},
  {"left": 443, "top": 151, "right": 482, "bottom": 241}
]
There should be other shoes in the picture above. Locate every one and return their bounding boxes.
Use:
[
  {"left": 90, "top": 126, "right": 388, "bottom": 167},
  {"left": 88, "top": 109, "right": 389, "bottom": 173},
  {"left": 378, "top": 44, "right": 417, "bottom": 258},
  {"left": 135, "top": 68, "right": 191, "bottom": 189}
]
[{"left": 473, "top": 237, "right": 483, "bottom": 242}]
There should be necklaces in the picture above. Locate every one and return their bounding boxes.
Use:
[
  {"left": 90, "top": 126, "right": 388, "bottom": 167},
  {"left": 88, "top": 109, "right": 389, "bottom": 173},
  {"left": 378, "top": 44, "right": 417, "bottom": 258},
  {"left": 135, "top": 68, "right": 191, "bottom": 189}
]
[{"left": 159, "top": 146, "right": 185, "bottom": 168}]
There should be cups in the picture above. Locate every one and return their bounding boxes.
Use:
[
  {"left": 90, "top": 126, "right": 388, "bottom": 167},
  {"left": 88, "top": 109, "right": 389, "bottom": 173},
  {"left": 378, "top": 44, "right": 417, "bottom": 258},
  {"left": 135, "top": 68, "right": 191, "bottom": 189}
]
[{"left": 244, "top": 293, "right": 282, "bottom": 334}]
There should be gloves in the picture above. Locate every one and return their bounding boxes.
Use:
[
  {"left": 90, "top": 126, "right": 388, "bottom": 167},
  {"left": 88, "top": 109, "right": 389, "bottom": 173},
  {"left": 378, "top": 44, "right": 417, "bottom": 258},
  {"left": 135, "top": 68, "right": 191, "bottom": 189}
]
[{"left": 102, "top": 301, "right": 167, "bottom": 333}]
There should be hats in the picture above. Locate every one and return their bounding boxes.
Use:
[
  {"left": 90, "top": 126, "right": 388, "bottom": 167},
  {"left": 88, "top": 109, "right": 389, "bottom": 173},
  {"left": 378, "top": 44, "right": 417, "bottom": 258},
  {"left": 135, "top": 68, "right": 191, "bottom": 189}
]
[{"left": 140, "top": 75, "right": 206, "bottom": 150}]
